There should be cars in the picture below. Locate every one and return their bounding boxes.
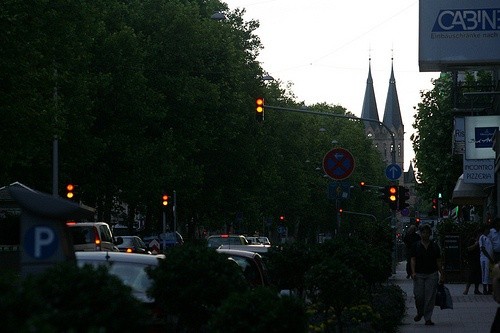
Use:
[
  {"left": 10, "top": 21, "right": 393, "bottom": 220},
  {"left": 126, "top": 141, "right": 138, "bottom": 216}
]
[{"left": 63, "top": 218, "right": 279, "bottom": 303}]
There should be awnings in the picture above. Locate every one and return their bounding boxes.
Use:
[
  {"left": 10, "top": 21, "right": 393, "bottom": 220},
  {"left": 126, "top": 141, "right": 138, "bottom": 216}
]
[{"left": 451, "top": 173, "right": 490, "bottom": 200}]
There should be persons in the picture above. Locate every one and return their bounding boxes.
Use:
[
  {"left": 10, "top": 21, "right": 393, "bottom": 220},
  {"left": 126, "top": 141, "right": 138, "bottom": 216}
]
[
  {"left": 461, "top": 223, "right": 500, "bottom": 333},
  {"left": 404, "top": 224, "right": 444, "bottom": 325}
]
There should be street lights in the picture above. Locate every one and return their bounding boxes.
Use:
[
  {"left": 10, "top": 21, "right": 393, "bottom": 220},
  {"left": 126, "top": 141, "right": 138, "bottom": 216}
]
[{"left": 256, "top": 98, "right": 265, "bottom": 121}]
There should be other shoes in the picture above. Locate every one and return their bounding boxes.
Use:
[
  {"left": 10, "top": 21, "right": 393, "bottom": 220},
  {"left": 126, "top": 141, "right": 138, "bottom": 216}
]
[
  {"left": 464, "top": 289, "right": 468, "bottom": 295},
  {"left": 483, "top": 291, "right": 488, "bottom": 295},
  {"left": 425, "top": 319, "right": 434, "bottom": 325},
  {"left": 414, "top": 313, "right": 422, "bottom": 321},
  {"left": 474, "top": 289, "right": 482, "bottom": 295}
]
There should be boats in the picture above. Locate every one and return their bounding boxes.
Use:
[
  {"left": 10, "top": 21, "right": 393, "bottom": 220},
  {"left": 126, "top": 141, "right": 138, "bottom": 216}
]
[{"left": 65, "top": 170, "right": 80, "bottom": 205}]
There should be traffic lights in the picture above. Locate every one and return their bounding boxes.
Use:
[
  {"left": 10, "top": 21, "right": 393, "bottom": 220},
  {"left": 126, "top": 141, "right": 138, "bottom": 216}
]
[
  {"left": 399, "top": 185, "right": 410, "bottom": 210},
  {"left": 340, "top": 208, "right": 344, "bottom": 213},
  {"left": 431, "top": 197, "right": 438, "bottom": 209},
  {"left": 278, "top": 209, "right": 288, "bottom": 222},
  {"left": 162, "top": 194, "right": 172, "bottom": 214},
  {"left": 388, "top": 185, "right": 398, "bottom": 208}
]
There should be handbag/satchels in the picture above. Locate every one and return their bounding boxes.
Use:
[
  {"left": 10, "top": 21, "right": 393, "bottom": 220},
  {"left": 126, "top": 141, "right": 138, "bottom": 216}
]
[{"left": 436, "top": 283, "right": 453, "bottom": 310}]
